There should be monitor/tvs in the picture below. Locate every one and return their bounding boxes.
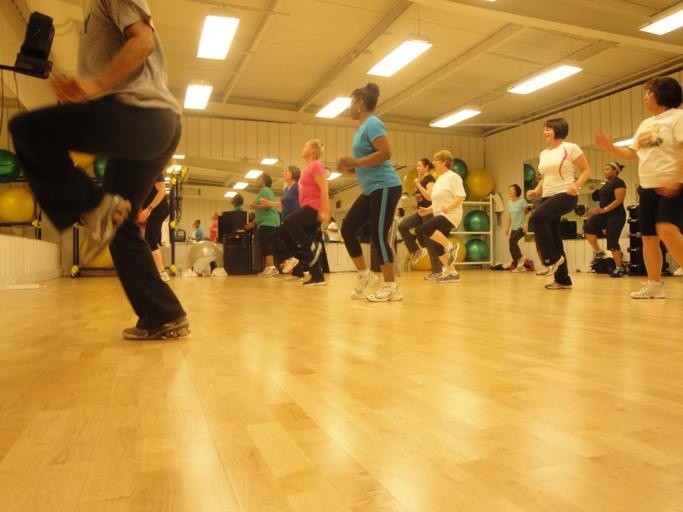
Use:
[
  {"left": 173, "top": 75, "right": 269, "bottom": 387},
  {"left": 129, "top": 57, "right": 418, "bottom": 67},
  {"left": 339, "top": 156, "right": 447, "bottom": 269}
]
[{"left": 21, "top": 10, "right": 57, "bottom": 58}]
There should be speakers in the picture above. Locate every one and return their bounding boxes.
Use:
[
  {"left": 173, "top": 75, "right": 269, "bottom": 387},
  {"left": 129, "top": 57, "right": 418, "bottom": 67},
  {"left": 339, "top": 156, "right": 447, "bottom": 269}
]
[{"left": 222, "top": 233, "right": 265, "bottom": 276}]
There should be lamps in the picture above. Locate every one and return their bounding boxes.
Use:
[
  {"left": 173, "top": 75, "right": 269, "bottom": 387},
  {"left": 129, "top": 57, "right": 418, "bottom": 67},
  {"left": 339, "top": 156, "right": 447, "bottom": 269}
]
[
  {"left": 639, "top": 2, "right": 683, "bottom": 36},
  {"left": 429, "top": 104, "right": 481, "bottom": 128},
  {"left": 366, "top": 4, "right": 433, "bottom": 78},
  {"left": 504, "top": 57, "right": 585, "bottom": 95}
]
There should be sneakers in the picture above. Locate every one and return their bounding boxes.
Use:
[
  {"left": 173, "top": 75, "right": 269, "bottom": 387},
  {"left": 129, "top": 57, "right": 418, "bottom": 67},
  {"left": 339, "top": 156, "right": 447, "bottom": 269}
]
[
  {"left": 410, "top": 250, "right": 426, "bottom": 265},
  {"left": 511, "top": 255, "right": 527, "bottom": 272},
  {"left": 536, "top": 255, "right": 565, "bottom": 277},
  {"left": 307, "top": 240, "right": 323, "bottom": 268},
  {"left": 257, "top": 266, "right": 280, "bottom": 277},
  {"left": 367, "top": 282, "right": 403, "bottom": 301},
  {"left": 79, "top": 193, "right": 132, "bottom": 264},
  {"left": 282, "top": 256, "right": 325, "bottom": 285},
  {"left": 610, "top": 266, "right": 625, "bottom": 277},
  {"left": 447, "top": 242, "right": 459, "bottom": 266},
  {"left": 545, "top": 282, "right": 573, "bottom": 289},
  {"left": 630, "top": 280, "right": 665, "bottom": 299},
  {"left": 424, "top": 271, "right": 460, "bottom": 282},
  {"left": 590, "top": 251, "right": 604, "bottom": 265},
  {"left": 351, "top": 269, "right": 379, "bottom": 299},
  {"left": 122, "top": 315, "right": 191, "bottom": 339}
]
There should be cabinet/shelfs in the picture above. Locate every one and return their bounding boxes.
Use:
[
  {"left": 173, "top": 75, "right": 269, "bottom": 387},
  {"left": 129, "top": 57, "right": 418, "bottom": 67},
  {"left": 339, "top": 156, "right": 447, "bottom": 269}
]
[
  {"left": 452, "top": 193, "right": 493, "bottom": 270},
  {"left": 0, "top": 175, "right": 41, "bottom": 239},
  {"left": 323, "top": 242, "right": 370, "bottom": 272}
]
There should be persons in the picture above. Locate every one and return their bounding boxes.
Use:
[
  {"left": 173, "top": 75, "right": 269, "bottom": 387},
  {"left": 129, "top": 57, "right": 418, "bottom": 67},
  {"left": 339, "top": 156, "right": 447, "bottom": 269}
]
[
  {"left": 8, "top": 0, "right": 189, "bottom": 340},
  {"left": 337, "top": 83, "right": 466, "bottom": 303},
  {"left": 505, "top": 76, "right": 683, "bottom": 299},
  {"left": 189, "top": 195, "right": 244, "bottom": 243},
  {"left": 249, "top": 139, "right": 337, "bottom": 286}
]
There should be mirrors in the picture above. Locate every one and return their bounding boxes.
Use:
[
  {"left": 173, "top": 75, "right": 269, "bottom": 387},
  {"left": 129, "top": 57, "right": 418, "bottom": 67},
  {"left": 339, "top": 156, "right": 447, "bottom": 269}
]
[{"left": 524, "top": 137, "right": 642, "bottom": 241}]
